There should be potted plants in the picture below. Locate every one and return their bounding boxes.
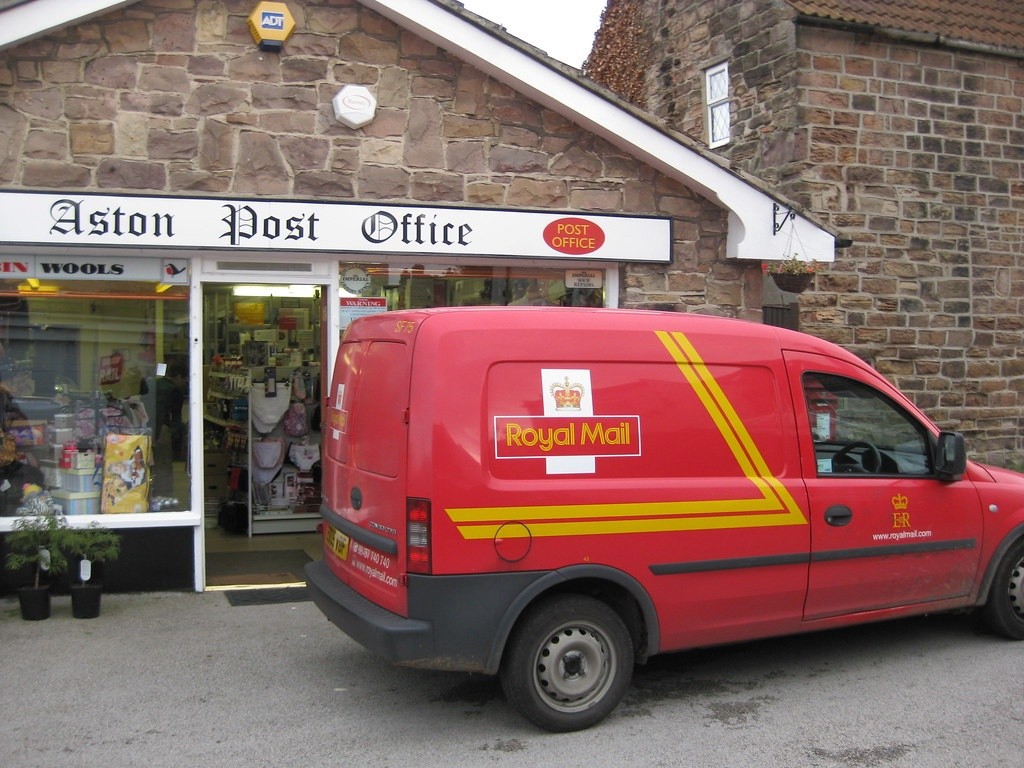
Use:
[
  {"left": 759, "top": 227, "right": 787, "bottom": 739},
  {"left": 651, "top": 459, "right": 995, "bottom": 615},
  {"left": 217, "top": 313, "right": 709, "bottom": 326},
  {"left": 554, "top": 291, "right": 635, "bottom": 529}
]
[
  {"left": 62, "top": 526, "right": 120, "bottom": 619},
  {"left": 4, "top": 510, "right": 64, "bottom": 621}
]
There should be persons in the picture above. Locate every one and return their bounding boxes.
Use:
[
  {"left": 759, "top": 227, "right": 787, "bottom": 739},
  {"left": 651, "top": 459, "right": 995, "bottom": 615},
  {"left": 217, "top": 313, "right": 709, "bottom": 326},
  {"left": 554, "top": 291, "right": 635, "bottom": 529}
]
[{"left": 161, "top": 352, "right": 184, "bottom": 428}]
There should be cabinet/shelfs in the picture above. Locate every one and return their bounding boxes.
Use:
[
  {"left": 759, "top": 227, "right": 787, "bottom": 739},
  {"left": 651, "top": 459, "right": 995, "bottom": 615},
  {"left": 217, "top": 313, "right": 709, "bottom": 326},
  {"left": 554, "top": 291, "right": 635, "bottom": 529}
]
[{"left": 203, "top": 370, "right": 245, "bottom": 428}]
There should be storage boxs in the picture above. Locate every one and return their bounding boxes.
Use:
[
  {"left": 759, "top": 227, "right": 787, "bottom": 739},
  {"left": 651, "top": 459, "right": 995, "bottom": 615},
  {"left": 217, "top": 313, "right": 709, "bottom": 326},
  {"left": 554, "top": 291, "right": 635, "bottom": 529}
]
[
  {"left": 49, "top": 428, "right": 72, "bottom": 443},
  {"left": 5, "top": 425, "right": 44, "bottom": 446},
  {"left": 59, "top": 450, "right": 78, "bottom": 469},
  {"left": 60, "top": 469, "right": 102, "bottom": 492},
  {"left": 205, "top": 476, "right": 225, "bottom": 498},
  {"left": 73, "top": 452, "right": 95, "bottom": 469},
  {"left": 49, "top": 491, "right": 101, "bottom": 515},
  {"left": 228, "top": 316, "right": 320, "bottom": 366},
  {"left": 39, "top": 459, "right": 60, "bottom": 488},
  {"left": 53, "top": 413, "right": 75, "bottom": 429},
  {"left": 204, "top": 450, "right": 224, "bottom": 475},
  {"left": 42, "top": 444, "right": 63, "bottom": 461}
]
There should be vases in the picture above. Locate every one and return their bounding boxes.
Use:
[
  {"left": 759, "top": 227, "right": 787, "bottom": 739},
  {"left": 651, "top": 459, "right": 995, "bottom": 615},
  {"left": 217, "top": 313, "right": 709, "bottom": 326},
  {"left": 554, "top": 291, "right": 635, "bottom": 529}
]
[{"left": 768, "top": 272, "right": 814, "bottom": 294}]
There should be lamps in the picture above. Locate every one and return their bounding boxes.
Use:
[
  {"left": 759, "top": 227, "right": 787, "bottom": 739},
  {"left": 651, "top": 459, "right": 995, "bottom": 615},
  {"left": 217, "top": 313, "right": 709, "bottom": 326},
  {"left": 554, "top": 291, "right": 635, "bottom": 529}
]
[
  {"left": 331, "top": 84, "right": 377, "bottom": 130},
  {"left": 156, "top": 283, "right": 172, "bottom": 292},
  {"left": 246, "top": 1, "right": 296, "bottom": 49},
  {"left": 28, "top": 279, "right": 39, "bottom": 287}
]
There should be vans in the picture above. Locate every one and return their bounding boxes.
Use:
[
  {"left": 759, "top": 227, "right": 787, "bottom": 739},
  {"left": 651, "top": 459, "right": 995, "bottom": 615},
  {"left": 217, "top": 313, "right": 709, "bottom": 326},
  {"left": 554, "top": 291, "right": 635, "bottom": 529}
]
[{"left": 307, "top": 305, "right": 1024, "bottom": 734}]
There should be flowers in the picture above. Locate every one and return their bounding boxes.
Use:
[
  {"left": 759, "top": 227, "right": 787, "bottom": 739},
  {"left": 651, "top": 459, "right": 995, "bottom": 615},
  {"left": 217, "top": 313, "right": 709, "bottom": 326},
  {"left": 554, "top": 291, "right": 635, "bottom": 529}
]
[{"left": 761, "top": 250, "right": 820, "bottom": 276}]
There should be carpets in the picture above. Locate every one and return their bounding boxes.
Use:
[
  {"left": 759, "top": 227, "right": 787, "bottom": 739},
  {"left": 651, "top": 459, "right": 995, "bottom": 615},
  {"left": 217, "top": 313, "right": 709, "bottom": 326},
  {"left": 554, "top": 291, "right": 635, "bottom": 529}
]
[
  {"left": 223, "top": 586, "right": 313, "bottom": 607},
  {"left": 206, "top": 549, "right": 313, "bottom": 587}
]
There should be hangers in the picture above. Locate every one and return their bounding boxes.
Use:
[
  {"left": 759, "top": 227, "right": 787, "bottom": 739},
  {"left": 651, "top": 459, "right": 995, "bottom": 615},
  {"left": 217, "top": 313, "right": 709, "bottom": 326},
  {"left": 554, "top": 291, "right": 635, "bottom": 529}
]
[{"left": 295, "top": 435, "right": 312, "bottom": 446}]
